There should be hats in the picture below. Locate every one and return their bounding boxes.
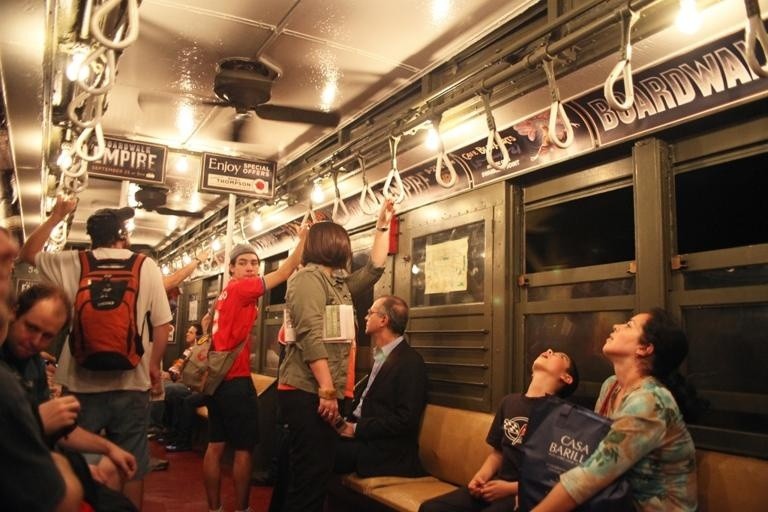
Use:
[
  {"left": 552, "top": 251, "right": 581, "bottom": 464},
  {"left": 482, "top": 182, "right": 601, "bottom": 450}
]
[
  {"left": 86, "top": 206, "right": 137, "bottom": 236},
  {"left": 229, "top": 243, "right": 262, "bottom": 264}
]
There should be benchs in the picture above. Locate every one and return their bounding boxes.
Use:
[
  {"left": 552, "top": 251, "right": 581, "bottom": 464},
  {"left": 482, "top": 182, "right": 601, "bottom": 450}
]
[
  {"left": 342, "top": 404, "right": 495, "bottom": 512},
  {"left": 196, "top": 373, "right": 277, "bottom": 417}
]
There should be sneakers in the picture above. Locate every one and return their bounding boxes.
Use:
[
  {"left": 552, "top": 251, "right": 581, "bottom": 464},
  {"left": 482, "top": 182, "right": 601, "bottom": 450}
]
[{"left": 144, "top": 431, "right": 192, "bottom": 472}]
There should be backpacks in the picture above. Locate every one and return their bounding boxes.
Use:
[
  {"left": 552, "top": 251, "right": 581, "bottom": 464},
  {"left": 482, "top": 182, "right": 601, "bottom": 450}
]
[{"left": 68, "top": 248, "right": 145, "bottom": 372}]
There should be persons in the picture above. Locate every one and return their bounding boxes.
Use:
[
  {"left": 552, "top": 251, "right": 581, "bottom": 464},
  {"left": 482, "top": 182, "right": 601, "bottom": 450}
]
[
  {"left": 0, "top": 228, "right": 96, "bottom": 512},
  {"left": 0, "top": 281, "right": 142, "bottom": 512},
  {"left": 511, "top": 305, "right": 700, "bottom": 511},
  {"left": 418, "top": 345, "right": 580, "bottom": 511},
  {"left": 250, "top": 296, "right": 429, "bottom": 487},
  {"left": 272, "top": 197, "right": 395, "bottom": 511},
  {"left": 19, "top": 195, "right": 173, "bottom": 510},
  {"left": 147, "top": 323, "right": 206, "bottom": 471},
  {"left": 200, "top": 222, "right": 312, "bottom": 512}
]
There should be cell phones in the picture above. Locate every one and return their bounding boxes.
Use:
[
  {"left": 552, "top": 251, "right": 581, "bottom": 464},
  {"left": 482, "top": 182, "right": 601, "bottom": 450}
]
[{"left": 331, "top": 414, "right": 347, "bottom": 434}]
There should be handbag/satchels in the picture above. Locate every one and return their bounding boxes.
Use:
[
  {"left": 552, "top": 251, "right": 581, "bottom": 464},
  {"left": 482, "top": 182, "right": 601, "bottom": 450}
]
[
  {"left": 514, "top": 394, "right": 634, "bottom": 512},
  {"left": 168, "top": 334, "right": 240, "bottom": 399},
  {"left": 278, "top": 269, "right": 357, "bottom": 349}
]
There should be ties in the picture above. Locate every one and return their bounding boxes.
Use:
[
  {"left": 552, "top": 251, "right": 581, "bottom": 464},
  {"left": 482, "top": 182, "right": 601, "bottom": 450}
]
[{"left": 352, "top": 353, "right": 386, "bottom": 418}]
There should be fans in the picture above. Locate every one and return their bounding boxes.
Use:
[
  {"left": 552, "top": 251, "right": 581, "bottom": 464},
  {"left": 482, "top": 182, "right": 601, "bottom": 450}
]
[
  {"left": 91, "top": 189, "right": 205, "bottom": 219},
  {"left": 137, "top": 71, "right": 340, "bottom": 141}
]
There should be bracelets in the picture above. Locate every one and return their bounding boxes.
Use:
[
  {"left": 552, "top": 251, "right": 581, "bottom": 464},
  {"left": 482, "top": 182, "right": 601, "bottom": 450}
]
[
  {"left": 375, "top": 225, "right": 389, "bottom": 233},
  {"left": 319, "top": 387, "right": 337, "bottom": 400}
]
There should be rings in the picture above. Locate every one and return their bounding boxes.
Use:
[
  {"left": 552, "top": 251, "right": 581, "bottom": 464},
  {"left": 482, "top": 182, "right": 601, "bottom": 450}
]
[{"left": 329, "top": 413, "right": 333, "bottom": 415}]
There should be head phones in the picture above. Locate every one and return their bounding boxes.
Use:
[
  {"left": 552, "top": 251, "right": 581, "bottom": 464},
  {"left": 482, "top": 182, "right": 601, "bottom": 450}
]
[{"left": 104, "top": 207, "right": 129, "bottom": 240}]
